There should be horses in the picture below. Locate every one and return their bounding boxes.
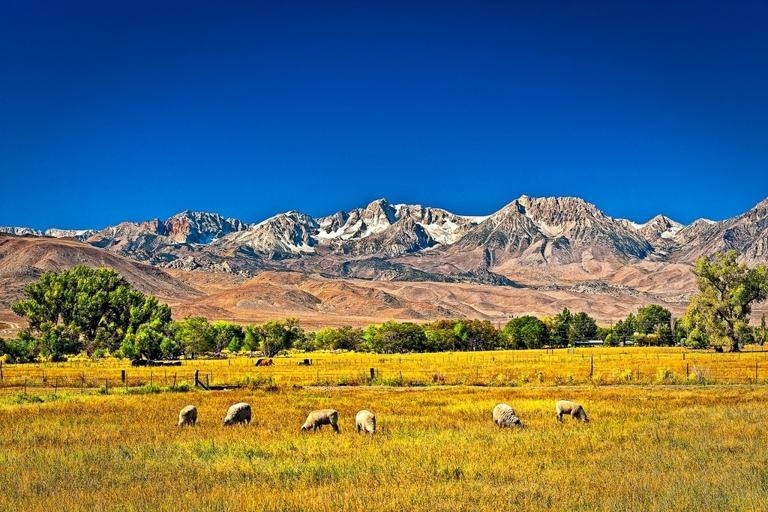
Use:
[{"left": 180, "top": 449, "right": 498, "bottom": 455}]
[{"left": 255, "top": 358, "right": 273, "bottom": 366}]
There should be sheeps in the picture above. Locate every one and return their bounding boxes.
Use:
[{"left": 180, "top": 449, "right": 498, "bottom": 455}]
[
  {"left": 355, "top": 409, "right": 376, "bottom": 434},
  {"left": 555, "top": 400, "right": 589, "bottom": 424},
  {"left": 177, "top": 404, "right": 198, "bottom": 426},
  {"left": 300, "top": 408, "right": 339, "bottom": 434},
  {"left": 493, "top": 403, "right": 522, "bottom": 430},
  {"left": 222, "top": 402, "right": 251, "bottom": 428}
]
